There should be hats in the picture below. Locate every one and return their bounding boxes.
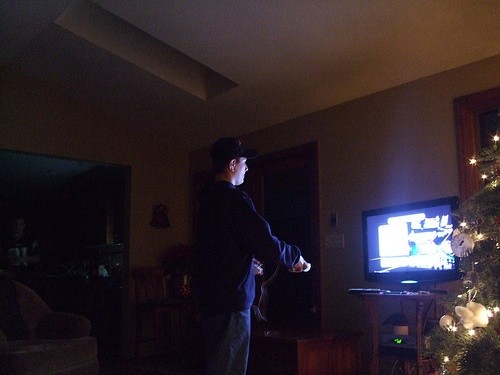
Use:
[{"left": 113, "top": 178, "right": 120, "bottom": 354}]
[{"left": 211, "top": 137, "right": 257, "bottom": 164}]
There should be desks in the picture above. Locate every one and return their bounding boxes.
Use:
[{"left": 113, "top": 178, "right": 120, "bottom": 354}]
[{"left": 358, "top": 291, "right": 449, "bottom": 375}]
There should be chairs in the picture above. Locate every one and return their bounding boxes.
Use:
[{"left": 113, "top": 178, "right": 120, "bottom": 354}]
[{"left": 132, "top": 267, "right": 165, "bottom": 358}]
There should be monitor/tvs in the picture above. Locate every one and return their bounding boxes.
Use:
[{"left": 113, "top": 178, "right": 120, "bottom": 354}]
[{"left": 361, "top": 196, "right": 462, "bottom": 292}]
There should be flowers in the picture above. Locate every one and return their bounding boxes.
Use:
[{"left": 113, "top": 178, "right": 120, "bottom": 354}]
[{"left": 162, "top": 244, "right": 191, "bottom": 269}]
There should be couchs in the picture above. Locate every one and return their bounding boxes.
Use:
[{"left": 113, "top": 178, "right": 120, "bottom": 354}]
[{"left": 0, "top": 280, "right": 100, "bottom": 375}]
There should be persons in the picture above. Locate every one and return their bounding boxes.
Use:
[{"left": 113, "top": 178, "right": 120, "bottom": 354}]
[
  {"left": 189, "top": 137, "right": 307, "bottom": 375},
  {"left": 0, "top": 211, "right": 38, "bottom": 270}
]
